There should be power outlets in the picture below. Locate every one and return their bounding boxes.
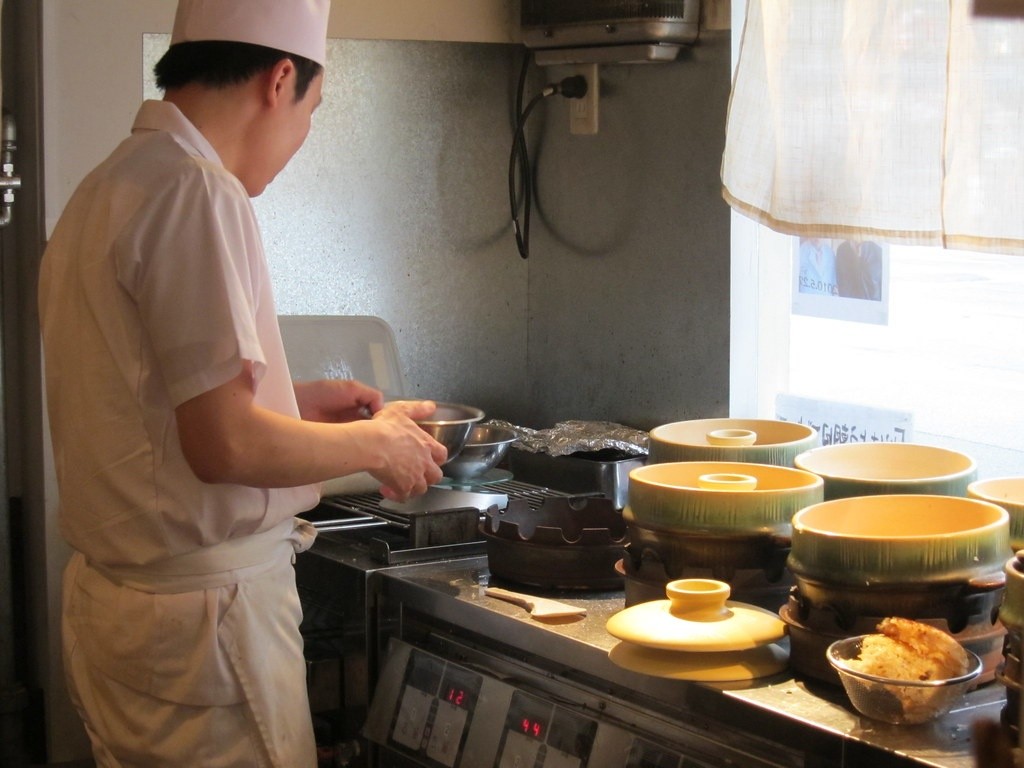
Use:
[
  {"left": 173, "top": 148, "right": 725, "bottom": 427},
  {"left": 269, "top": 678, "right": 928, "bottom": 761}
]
[{"left": 566, "top": 63, "right": 598, "bottom": 136}]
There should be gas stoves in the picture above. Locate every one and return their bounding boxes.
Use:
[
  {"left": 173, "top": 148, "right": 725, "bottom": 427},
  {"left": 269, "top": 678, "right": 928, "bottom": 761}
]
[{"left": 371, "top": 539, "right": 1023, "bottom": 767}]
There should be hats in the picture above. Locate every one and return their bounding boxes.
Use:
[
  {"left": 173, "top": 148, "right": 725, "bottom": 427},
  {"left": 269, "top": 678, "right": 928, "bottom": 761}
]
[{"left": 169, "top": 0, "right": 331, "bottom": 69}]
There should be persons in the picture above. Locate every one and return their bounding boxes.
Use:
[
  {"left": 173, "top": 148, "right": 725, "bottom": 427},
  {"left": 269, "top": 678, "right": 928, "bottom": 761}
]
[{"left": 38, "top": 0, "right": 447, "bottom": 768}]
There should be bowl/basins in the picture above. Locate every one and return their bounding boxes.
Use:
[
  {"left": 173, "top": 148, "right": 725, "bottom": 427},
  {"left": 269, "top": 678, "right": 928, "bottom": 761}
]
[
  {"left": 445, "top": 426, "right": 520, "bottom": 480},
  {"left": 826, "top": 634, "right": 983, "bottom": 726},
  {"left": 356, "top": 401, "right": 485, "bottom": 464}
]
